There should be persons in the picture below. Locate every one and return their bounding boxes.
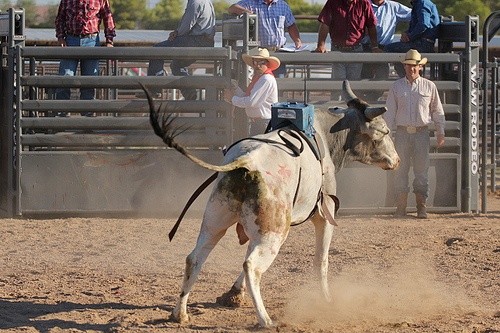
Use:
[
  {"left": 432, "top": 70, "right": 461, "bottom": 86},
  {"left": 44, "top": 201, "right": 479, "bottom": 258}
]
[
  {"left": 383, "top": 49, "right": 445, "bottom": 218},
  {"left": 227, "top": 0, "right": 301, "bottom": 78},
  {"left": 56, "top": 0, "right": 117, "bottom": 116},
  {"left": 136, "top": 0, "right": 215, "bottom": 101},
  {"left": 312, "top": 0, "right": 440, "bottom": 102},
  {"left": 224, "top": 47, "right": 281, "bottom": 136}
]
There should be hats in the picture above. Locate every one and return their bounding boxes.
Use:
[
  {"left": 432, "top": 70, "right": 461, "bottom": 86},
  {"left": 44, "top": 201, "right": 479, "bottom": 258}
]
[
  {"left": 399, "top": 49, "right": 427, "bottom": 65},
  {"left": 242, "top": 47, "right": 280, "bottom": 70}
]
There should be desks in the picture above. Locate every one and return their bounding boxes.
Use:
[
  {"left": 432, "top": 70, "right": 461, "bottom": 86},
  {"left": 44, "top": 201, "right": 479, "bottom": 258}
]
[{"left": 438, "top": 21, "right": 476, "bottom": 78}]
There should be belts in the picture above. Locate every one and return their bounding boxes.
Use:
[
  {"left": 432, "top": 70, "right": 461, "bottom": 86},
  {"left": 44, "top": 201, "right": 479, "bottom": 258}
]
[
  {"left": 363, "top": 43, "right": 384, "bottom": 49},
  {"left": 331, "top": 44, "right": 361, "bottom": 50},
  {"left": 248, "top": 117, "right": 262, "bottom": 123},
  {"left": 68, "top": 33, "right": 98, "bottom": 39},
  {"left": 267, "top": 46, "right": 279, "bottom": 51},
  {"left": 420, "top": 37, "right": 435, "bottom": 44},
  {"left": 397, "top": 125, "right": 428, "bottom": 134}
]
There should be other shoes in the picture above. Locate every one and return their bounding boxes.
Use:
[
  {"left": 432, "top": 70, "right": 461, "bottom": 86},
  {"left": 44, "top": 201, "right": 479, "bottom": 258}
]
[
  {"left": 84, "top": 111, "right": 96, "bottom": 117},
  {"left": 134, "top": 91, "right": 162, "bottom": 99},
  {"left": 186, "top": 91, "right": 197, "bottom": 101},
  {"left": 58, "top": 112, "right": 71, "bottom": 116}
]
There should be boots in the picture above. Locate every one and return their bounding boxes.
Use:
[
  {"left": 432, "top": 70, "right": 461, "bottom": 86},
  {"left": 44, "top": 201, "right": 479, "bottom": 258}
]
[
  {"left": 416, "top": 192, "right": 428, "bottom": 218},
  {"left": 394, "top": 192, "right": 408, "bottom": 217}
]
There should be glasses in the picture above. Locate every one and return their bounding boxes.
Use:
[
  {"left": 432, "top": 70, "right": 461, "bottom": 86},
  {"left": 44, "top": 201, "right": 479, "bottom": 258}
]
[{"left": 253, "top": 60, "right": 268, "bottom": 66}]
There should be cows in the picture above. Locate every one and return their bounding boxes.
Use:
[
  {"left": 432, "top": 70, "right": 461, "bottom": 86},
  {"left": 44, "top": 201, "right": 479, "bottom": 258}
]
[{"left": 139, "top": 79, "right": 400, "bottom": 330}]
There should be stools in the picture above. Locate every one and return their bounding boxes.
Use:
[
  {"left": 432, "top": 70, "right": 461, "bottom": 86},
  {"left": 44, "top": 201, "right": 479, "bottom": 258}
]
[{"left": 423, "top": 46, "right": 437, "bottom": 80}]
